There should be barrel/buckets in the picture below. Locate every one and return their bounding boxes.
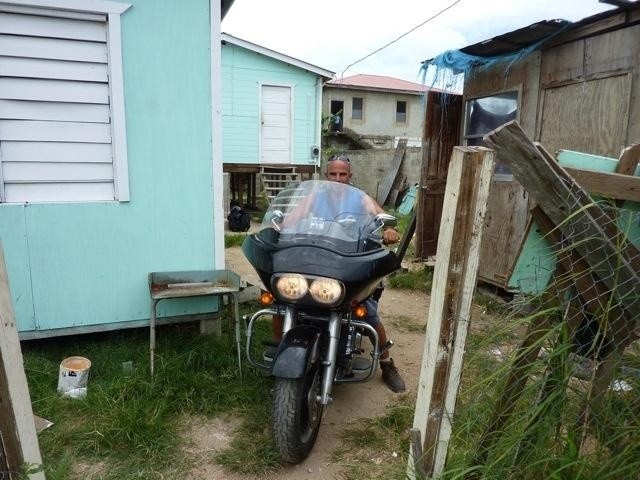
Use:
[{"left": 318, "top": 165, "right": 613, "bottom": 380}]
[{"left": 56, "top": 356, "right": 92, "bottom": 395}]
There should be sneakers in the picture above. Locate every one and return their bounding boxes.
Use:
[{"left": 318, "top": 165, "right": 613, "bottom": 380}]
[{"left": 379, "top": 357, "right": 405, "bottom": 394}]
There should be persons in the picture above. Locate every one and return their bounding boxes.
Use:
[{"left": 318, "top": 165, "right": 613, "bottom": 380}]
[
  {"left": 335, "top": 115, "right": 341, "bottom": 130},
  {"left": 261, "top": 153, "right": 406, "bottom": 393}
]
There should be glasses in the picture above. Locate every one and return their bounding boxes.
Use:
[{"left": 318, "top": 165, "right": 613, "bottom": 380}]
[{"left": 327, "top": 155, "right": 351, "bottom": 162}]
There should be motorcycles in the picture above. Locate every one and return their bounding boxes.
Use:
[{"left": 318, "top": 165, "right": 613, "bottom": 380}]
[{"left": 239, "top": 182, "right": 403, "bottom": 466}]
